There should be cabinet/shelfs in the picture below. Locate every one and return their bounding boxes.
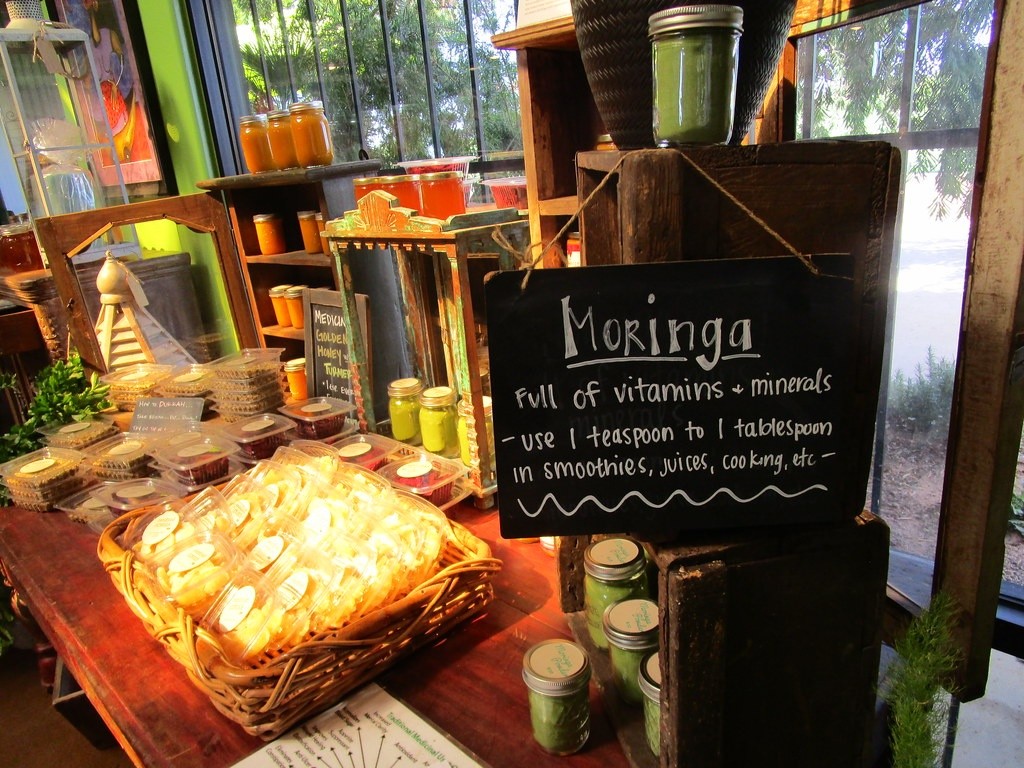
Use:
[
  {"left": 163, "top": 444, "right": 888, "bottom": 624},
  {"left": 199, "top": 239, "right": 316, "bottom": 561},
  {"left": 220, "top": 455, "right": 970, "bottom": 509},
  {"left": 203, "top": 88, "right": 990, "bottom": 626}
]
[
  {"left": 36, "top": 156, "right": 415, "bottom": 424},
  {"left": 556, "top": 514, "right": 891, "bottom": 768},
  {"left": 491, "top": 19, "right": 899, "bottom": 269}
]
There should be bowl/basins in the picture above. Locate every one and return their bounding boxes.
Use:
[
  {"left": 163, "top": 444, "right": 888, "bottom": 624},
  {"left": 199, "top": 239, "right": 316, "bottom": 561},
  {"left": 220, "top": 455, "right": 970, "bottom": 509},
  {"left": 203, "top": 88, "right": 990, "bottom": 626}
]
[
  {"left": 477, "top": 176, "right": 528, "bottom": 210},
  {"left": 396, "top": 156, "right": 482, "bottom": 209},
  {"left": 331, "top": 433, "right": 405, "bottom": 471},
  {"left": 0, "top": 354, "right": 357, "bottom": 534},
  {"left": 375, "top": 454, "right": 470, "bottom": 507}
]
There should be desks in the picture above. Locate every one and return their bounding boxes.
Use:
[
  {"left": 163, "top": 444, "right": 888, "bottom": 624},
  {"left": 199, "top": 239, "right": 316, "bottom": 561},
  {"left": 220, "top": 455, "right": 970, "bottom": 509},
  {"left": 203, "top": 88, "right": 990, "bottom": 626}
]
[{"left": 0, "top": 504, "right": 631, "bottom": 768}]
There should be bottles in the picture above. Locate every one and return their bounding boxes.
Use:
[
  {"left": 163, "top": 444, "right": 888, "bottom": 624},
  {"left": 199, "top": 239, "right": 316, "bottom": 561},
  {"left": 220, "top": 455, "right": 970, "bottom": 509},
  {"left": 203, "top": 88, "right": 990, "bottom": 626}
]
[
  {"left": 584, "top": 539, "right": 647, "bottom": 650},
  {"left": 268, "top": 284, "right": 307, "bottom": 328},
  {"left": 253, "top": 212, "right": 285, "bottom": 254},
  {"left": 650, "top": 4, "right": 744, "bottom": 147},
  {"left": 299, "top": 211, "right": 329, "bottom": 256},
  {"left": 387, "top": 377, "right": 495, "bottom": 468},
  {"left": 603, "top": 599, "right": 659, "bottom": 709},
  {"left": 353, "top": 172, "right": 466, "bottom": 220},
  {"left": 639, "top": 652, "right": 664, "bottom": 759},
  {"left": 565, "top": 231, "right": 582, "bottom": 267},
  {"left": 596, "top": 134, "right": 620, "bottom": 150},
  {"left": 284, "top": 358, "right": 308, "bottom": 399},
  {"left": 522, "top": 639, "right": 591, "bottom": 756},
  {"left": 240, "top": 101, "right": 333, "bottom": 174}
]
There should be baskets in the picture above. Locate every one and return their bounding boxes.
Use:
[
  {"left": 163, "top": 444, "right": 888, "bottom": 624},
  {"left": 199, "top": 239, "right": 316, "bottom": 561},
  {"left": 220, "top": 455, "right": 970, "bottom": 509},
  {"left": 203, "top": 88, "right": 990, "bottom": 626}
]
[
  {"left": 571, "top": 0, "right": 798, "bottom": 149},
  {"left": 98, "top": 447, "right": 501, "bottom": 742}
]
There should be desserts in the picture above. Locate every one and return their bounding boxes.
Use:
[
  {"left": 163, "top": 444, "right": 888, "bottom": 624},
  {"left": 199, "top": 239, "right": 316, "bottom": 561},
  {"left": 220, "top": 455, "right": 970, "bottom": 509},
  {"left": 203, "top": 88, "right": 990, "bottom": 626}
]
[{"left": 4, "top": 357, "right": 454, "bottom": 523}]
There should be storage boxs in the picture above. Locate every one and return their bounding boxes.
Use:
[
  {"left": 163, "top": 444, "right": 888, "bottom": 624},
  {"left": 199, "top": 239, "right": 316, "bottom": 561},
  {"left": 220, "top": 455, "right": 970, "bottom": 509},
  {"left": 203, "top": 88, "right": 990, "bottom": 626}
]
[{"left": 1, "top": 348, "right": 474, "bottom": 536}]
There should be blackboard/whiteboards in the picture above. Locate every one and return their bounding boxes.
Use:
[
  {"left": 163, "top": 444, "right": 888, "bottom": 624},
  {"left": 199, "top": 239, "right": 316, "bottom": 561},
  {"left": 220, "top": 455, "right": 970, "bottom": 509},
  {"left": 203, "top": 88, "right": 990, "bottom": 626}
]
[
  {"left": 485, "top": 254, "right": 890, "bottom": 540},
  {"left": 301, "top": 288, "right": 371, "bottom": 426}
]
